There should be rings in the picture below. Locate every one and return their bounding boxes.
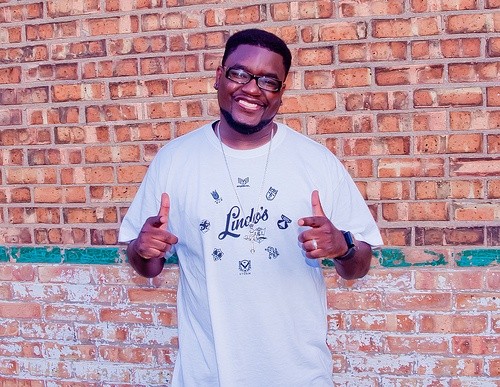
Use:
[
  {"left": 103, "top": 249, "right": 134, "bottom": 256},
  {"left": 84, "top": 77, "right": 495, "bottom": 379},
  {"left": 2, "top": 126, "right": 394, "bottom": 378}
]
[{"left": 310, "top": 238, "right": 319, "bottom": 251}]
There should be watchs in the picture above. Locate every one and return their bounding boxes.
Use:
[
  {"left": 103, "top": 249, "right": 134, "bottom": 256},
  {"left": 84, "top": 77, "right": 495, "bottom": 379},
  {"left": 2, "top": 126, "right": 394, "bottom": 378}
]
[{"left": 336, "top": 229, "right": 359, "bottom": 265}]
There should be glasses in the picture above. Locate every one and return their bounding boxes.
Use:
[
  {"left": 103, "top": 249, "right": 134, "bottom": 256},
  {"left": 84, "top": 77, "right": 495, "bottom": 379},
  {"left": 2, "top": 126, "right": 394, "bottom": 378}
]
[{"left": 222, "top": 65, "right": 285, "bottom": 93}]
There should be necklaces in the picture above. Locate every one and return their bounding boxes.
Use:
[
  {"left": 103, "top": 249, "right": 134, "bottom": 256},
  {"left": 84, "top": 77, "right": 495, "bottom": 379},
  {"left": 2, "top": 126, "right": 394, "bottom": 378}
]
[{"left": 216, "top": 120, "right": 275, "bottom": 253}]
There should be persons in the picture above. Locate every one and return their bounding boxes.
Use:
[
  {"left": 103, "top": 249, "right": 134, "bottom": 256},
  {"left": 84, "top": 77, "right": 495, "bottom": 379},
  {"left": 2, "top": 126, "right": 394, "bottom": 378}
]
[{"left": 118, "top": 28, "right": 386, "bottom": 384}]
